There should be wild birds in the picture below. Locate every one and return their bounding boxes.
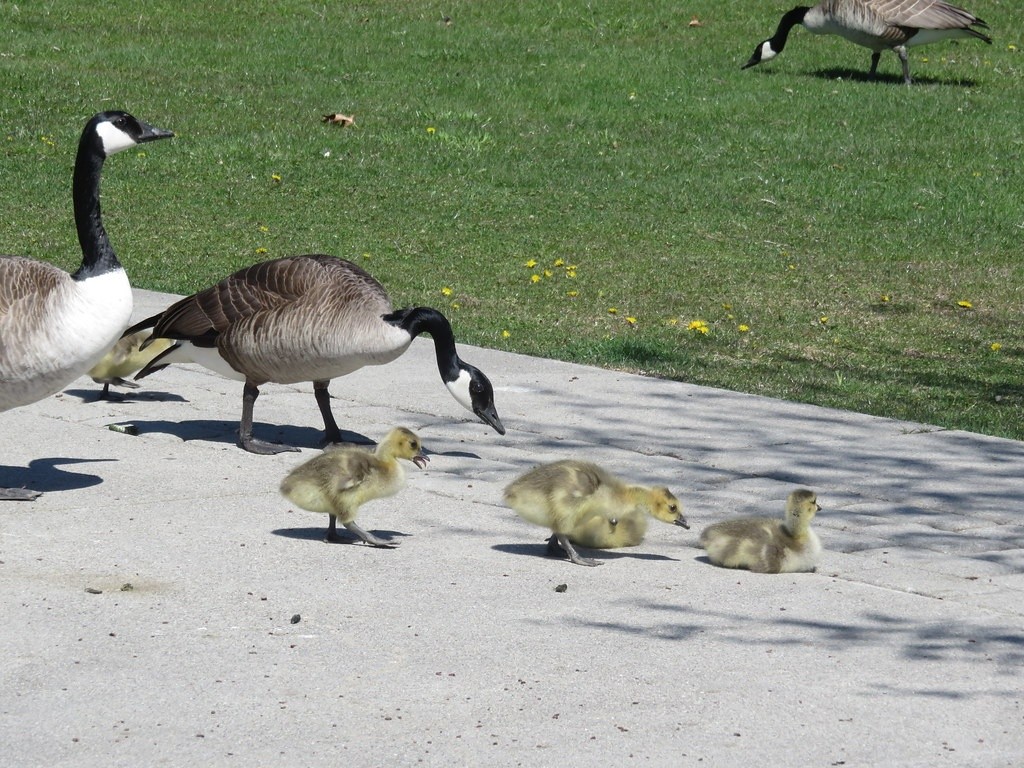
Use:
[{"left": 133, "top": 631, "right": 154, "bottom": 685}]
[
  {"left": 699, "top": 488, "right": 822, "bottom": 574},
  {"left": 279, "top": 426, "right": 431, "bottom": 545},
  {"left": 120, "top": 255, "right": 506, "bottom": 455},
  {"left": 741, "top": 0, "right": 992, "bottom": 87},
  {"left": 88, "top": 329, "right": 175, "bottom": 401},
  {"left": 0, "top": 110, "right": 175, "bottom": 501},
  {"left": 501, "top": 459, "right": 690, "bottom": 567}
]
